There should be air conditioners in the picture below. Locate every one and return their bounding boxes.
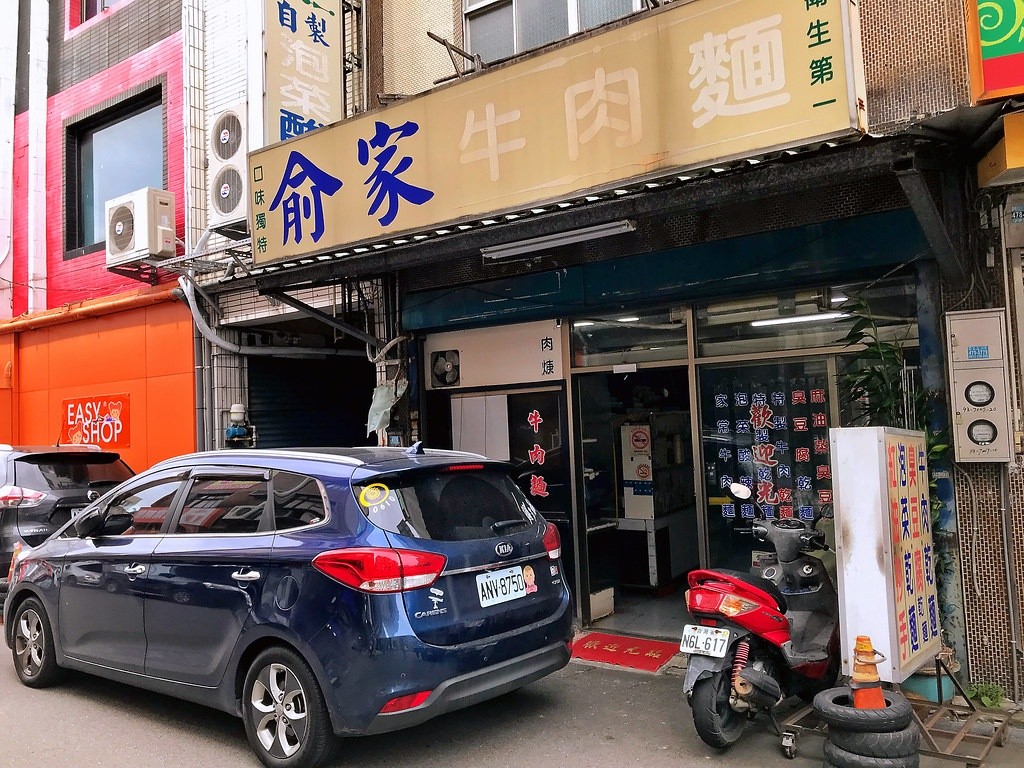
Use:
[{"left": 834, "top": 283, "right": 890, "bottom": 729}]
[
  {"left": 103, "top": 186, "right": 175, "bottom": 270},
  {"left": 207, "top": 101, "right": 247, "bottom": 234}
]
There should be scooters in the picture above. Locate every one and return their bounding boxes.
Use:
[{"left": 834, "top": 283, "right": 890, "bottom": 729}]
[{"left": 680, "top": 481, "right": 840, "bottom": 749}]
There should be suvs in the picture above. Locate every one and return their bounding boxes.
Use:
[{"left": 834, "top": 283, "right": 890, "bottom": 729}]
[{"left": 0, "top": 442, "right": 141, "bottom": 623}]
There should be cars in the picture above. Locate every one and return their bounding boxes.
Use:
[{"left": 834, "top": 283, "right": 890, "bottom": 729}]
[{"left": 3, "top": 441, "right": 575, "bottom": 768}]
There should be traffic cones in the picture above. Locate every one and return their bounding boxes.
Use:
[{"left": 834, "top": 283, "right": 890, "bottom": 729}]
[{"left": 847, "top": 636, "right": 888, "bottom": 709}]
